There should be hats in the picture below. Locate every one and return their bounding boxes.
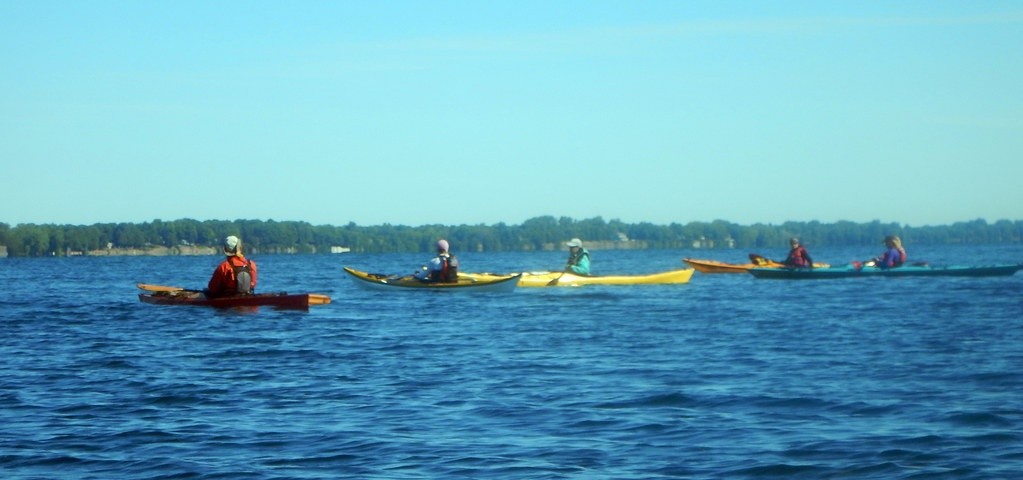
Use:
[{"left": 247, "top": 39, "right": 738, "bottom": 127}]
[
  {"left": 566, "top": 238, "right": 582, "bottom": 248},
  {"left": 790, "top": 238, "right": 798, "bottom": 244},
  {"left": 437, "top": 240, "right": 449, "bottom": 252},
  {"left": 223, "top": 236, "right": 241, "bottom": 256}
]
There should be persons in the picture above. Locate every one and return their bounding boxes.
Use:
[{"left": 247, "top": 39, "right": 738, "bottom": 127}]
[
  {"left": 413, "top": 239, "right": 458, "bottom": 284},
  {"left": 208, "top": 236, "right": 257, "bottom": 295},
  {"left": 873, "top": 235, "right": 906, "bottom": 271},
  {"left": 565, "top": 238, "right": 591, "bottom": 276},
  {"left": 780, "top": 238, "right": 813, "bottom": 269}
]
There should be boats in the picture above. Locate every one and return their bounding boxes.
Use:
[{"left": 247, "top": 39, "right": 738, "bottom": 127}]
[
  {"left": 747, "top": 255, "right": 1023, "bottom": 278},
  {"left": 683, "top": 254, "right": 879, "bottom": 275},
  {"left": 138, "top": 289, "right": 333, "bottom": 309},
  {"left": 343, "top": 263, "right": 523, "bottom": 293},
  {"left": 422, "top": 258, "right": 697, "bottom": 287}
]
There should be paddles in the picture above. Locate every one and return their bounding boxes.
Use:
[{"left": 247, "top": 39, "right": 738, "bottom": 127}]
[
  {"left": 545, "top": 265, "right": 571, "bottom": 286},
  {"left": 136, "top": 282, "right": 205, "bottom": 294}
]
